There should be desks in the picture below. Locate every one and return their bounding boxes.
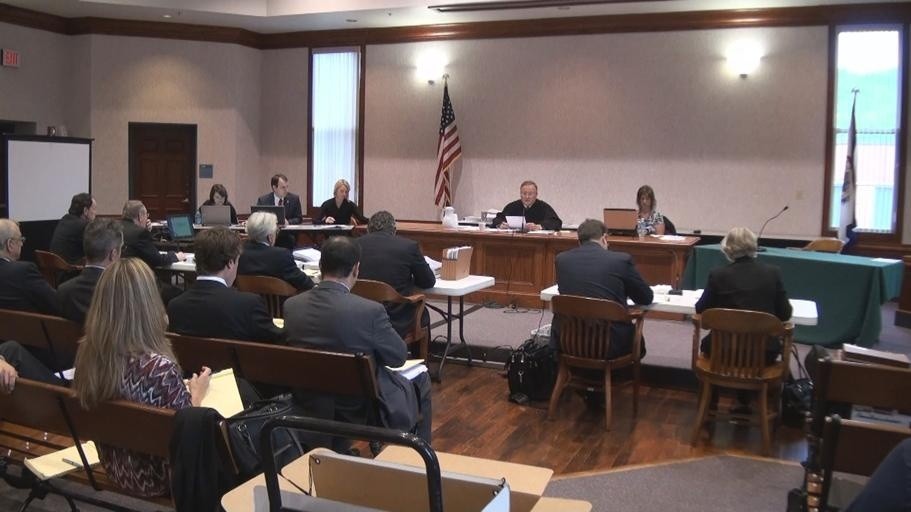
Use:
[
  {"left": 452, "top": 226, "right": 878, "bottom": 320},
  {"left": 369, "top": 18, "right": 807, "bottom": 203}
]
[
  {"left": 680, "top": 242, "right": 905, "bottom": 351},
  {"left": 538, "top": 276, "right": 821, "bottom": 391},
  {"left": 151, "top": 248, "right": 496, "bottom": 380},
  {"left": 151, "top": 218, "right": 700, "bottom": 324}
]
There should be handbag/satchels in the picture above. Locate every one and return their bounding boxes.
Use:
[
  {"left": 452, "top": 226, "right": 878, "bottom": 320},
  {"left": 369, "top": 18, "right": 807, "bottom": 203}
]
[
  {"left": 503, "top": 338, "right": 552, "bottom": 403},
  {"left": 781, "top": 343, "right": 817, "bottom": 429},
  {"left": 225, "top": 393, "right": 304, "bottom": 474}
]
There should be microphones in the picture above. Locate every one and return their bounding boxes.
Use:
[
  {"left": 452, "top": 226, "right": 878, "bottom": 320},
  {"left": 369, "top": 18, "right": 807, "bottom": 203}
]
[{"left": 757, "top": 206, "right": 789, "bottom": 252}]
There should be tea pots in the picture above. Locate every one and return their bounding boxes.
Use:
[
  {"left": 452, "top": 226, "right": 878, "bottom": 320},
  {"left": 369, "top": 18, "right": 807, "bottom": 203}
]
[{"left": 440, "top": 206, "right": 458, "bottom": 229}]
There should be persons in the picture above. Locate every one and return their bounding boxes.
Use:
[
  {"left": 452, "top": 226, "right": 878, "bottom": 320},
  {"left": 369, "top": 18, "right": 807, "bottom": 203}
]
[
  {"left": 73, "top": 258, "right": 299, "bottom": 499},
  {"left": 610, "top": 185, "right": 676, "bottom": 235},
  {"left": 843, "top": 438, "right": 911, "bottom": 512},
  {"left": 491, "top": 180, "right": 563, "bottom": 232},
  {"left": 554, "top": 218, "right": 654, "bottom": 409},
  {"left": 695, "top": 223, "right": 792, "bottom": 414},
  {"left": 1, "top": 172, "right": 436, "bottom": 455}
]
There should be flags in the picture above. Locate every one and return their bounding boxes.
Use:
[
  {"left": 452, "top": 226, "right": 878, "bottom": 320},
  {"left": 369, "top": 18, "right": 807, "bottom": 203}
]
[
  {"left": 838, "top": 94, "right": 858, "bottom": 254},
  {"left": 433, "top": 76, "right": 462, "bottom": 212}
]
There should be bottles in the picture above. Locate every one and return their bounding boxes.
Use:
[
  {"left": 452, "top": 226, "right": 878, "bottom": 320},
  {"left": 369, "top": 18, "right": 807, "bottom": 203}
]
[
  {"left": 195, "top": 208, "right": 202, "bottom": 226},
  {"left": 639, "top": 218, "right": 645, "bottom": 240}
]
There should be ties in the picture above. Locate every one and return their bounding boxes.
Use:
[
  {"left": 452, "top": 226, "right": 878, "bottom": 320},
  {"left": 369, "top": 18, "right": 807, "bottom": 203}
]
[{"left": 277, "top": 198, "right": 282, "bottom": 206}]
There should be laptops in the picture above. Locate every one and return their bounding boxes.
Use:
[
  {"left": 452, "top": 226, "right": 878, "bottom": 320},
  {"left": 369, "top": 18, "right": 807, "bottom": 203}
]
[
  {"left": 603, "top": 208, "right": 637, "bottom": 236},
  {"left": 167, "top": 214, "right": 195, "bottom": 242},
  {"left": 201, "top": 205, "right": 231, "bottom": 227},
  {"left": 251, "top": 206, "right": 285, "bottom": 226}
]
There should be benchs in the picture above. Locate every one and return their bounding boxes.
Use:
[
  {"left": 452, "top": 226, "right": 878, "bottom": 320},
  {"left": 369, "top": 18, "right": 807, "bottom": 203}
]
[
  {"left": 785, "top": 344, "right": 908, "bottom": 508},
  {"left": 2, "top": 309, "right": 420, "bottom": 510}
]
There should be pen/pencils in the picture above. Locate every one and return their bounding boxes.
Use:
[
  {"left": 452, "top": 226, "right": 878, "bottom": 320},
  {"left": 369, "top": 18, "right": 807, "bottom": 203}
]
[{"left": 63, "top": 458, "right": 83, "bottom": 468}]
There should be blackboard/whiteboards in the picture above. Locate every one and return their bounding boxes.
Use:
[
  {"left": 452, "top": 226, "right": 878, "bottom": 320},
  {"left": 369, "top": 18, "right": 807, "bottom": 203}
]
[{"left": 360, "top": 15, "right": 836, "bottom": 248}]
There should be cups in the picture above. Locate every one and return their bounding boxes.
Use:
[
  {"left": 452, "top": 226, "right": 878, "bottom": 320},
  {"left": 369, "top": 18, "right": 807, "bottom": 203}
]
[
  {"left": 479, "top": 223, "right": 486, "bottom": 231},
  {"left": 48, "top": 127, "right": 55, "bottom": 136},
  {"left": 56, "top": 127, "right": 65, "bottom": 136}
]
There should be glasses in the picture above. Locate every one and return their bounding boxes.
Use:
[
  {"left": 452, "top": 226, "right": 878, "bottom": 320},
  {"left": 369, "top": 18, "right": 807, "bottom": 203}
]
[{"left": 8, "top": 236, "right": 25, "bottom": 244}]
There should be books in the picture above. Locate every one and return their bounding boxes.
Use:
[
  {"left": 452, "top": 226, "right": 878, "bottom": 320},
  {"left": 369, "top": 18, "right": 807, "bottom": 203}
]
[{"left": 24, "top": 440, "right": 99, "bottom": 481}]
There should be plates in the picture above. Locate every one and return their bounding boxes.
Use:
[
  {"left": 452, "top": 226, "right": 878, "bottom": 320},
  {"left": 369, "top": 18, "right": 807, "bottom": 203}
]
[{"left": 527, "top": 231, "right": 555, "bottom": 236}]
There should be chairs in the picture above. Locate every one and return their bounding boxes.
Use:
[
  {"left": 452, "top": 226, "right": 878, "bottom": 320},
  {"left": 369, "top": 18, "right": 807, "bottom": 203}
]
[
  {"left": 688, "top": 307, "right": 793, "bottom": 449},
  {"left": 35, "top": 248, "right": 71, "bottom": 271},
  {"left": 547, "top": 296, "right": 645, "bottom": 430},
  {"left": 349, "top": 280, "right": 429, "bottom": 369},
  {"left": 234, "top": 275, "right": 297, "bottom": 318}
]
